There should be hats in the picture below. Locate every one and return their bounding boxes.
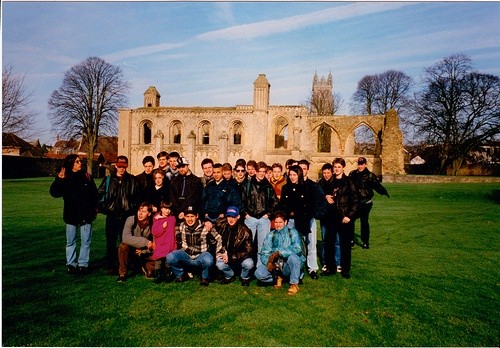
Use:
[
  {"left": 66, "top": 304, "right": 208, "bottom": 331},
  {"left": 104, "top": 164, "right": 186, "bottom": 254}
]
[
  {"left": 184, "top": 205, "right": 198, "bottom": 218},
  {"left": 357, "top": 157, "right": 366, "bottom": 163},
  {"left": 225, "top": 206, "right": 240, "bottom": 218},
  {"left": 175, "top": 157, "right": 190, "bottom": 167},
  {"left": 116, "top": 162, "right": 127, "bottom": 168}
]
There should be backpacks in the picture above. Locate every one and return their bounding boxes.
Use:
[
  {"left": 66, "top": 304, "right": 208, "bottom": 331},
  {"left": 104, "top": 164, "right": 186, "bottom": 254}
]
[{"left": 98, "top": 175, "right": 112, "bottom": 215}]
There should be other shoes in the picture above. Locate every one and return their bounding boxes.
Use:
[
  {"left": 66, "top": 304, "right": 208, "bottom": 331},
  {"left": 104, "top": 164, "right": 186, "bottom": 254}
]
[
  {"left": 200, "top": 278, "right": 209, "bottom": 286},
  {"left": 65, "top": 260, "right": 174, "bottom": 283},
  {"left": 342, "top": 272, "right": 350, "bottom": 279},
  {"left": 321, "top": 265, "right": 327, "bottom": 271},
  {"left": 310, "top": 271, "right": 318, "bottom": 279},
  {"left": 187, "top": 272, "right": 193, "bottom": 279},
  {"left": 337, "top": 265, "right": 342, "bottom": 272},
  {"left": 350, "top": 237, "right": 354, "bottom": 247},
  {"left": 176, "top": 274, "right": 185, "bottom": 282},
  {"left": 220, "top": 273, "right": 236, "bottom": 284},
  {"left": 287, "top": 284, "right": 299, "bottom": 296},
  {"left": 299, "top": 278, "right": 303, "bottom": 284},
  {"left": 320, "top": 269, "right": 336, "bottom": 276},
  {"left": 241, "top": 277, "right": 250, "bottom": 286},
  {"left": 362, "top": 241, "right": 369, "bottom": 249}
]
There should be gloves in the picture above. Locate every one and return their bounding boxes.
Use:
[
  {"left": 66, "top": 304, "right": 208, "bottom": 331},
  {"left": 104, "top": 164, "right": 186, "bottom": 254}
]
[
  {"left": 381, "top": 191, "right": 389, "bottom": 198},
  {"left": 269, "top": 251, "right": 279, "bottom": 263},
  {"left": 268, "top": 262, "right": 276, "bottom": 271}
]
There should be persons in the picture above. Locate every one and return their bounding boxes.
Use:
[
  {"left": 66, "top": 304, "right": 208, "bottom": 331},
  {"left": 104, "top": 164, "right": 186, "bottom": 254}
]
[
  {"left": 253, "top": 210, "right": 307, "bottom": 296},
  {"left": 127, "top": 150, "right": 204, "bottom": 223},
  {"left": 116, "top": 202, "right": 157, "bottom": 281},
  {"left": 165, "top": 206, "right": 222, "bottom": 286},
  {"left": 205, "top": 206, "right": 258, "bottom": 281},
  {"left": 276, "top": 158, "right": 325, "bottom": 285},
  {"left": 201, "top": 158, "right": 248, "bottom": 217},
  {"left": 347, "top": 157, "right": 390, "bottom": 249},
  {"left": 241, "top": 159, "right": 288, "bottom": 230},
  {"left": 49, "top": 154, "right": 99, "bottom": 272},
  {"left": 149, "top": 201, "right": 178, "bottom": 283},
  {"left": 317, "top": 158, "right": 358, "bottom": 279},
  {"left": 95, "top": 156, "right": 137, "bottom": 268}
]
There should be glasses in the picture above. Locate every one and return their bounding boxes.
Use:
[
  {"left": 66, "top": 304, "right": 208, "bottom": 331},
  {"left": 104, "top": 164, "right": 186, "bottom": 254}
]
[
  {"left": 235, "top": 168, "right": 245, "bottom": 172},
  {"left": 358, "top": 163, "right": 364, "bottom": 165},
  {"left": 177, "top": 164, "right": 185, "bottom": 168},
  {"left": 74, "top": 159, "right": 82, "bottom": 164}
]
